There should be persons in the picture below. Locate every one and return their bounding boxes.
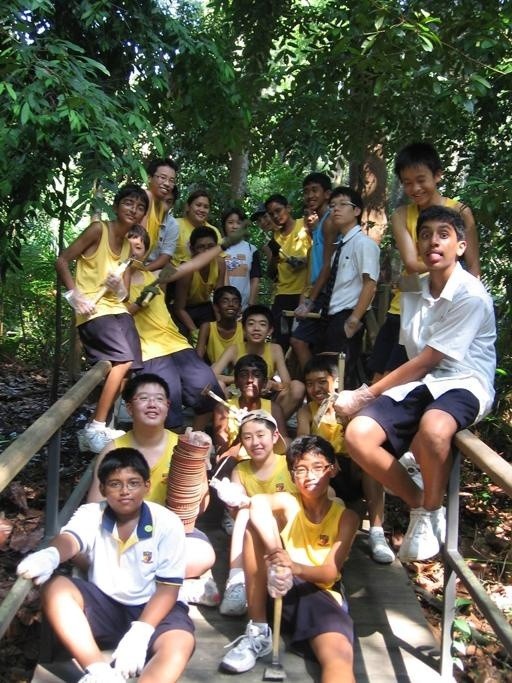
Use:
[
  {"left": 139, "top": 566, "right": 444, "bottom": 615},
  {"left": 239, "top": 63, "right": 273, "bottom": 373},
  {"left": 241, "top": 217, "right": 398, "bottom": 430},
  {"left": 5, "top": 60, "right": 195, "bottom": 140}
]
[
  {"left": 295, "top": 356, "right": 396, "bottom": 563},
  {"left": 335, "top": 209, "right": 495, "bottom": 560},
  {"left": 85, "top": 372, "right": 222, "bottom": 605},
  {"left": 58, "top": 157, "right": 380, "bottom": 533},
  {"left": 215, "top": 434, "right": 359, "bottom": 681},
  {"left": 209, "top": 409, "right": 297, "bottom": 614},
  {"left": 14, "top": 446, "right": 198, "bottom": 683}
]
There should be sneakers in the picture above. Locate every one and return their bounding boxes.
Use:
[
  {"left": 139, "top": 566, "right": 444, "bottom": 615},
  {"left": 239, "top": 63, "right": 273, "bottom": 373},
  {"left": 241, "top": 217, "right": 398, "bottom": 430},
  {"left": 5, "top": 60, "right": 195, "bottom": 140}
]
[
  {"left": 369, "top": 531, "right": 396, "bottom": 563},
  {"left": 399, "top": 507, "right": 447, "bottom": 560},
  {"left": 78, "top": 430, "right": 124, "bottom": 453},
  {"left": 220, "top": 622, "right": 274, "bottom": 672},
  {"left": 220, "top": 579, "right": 249, "bottom": 615}
]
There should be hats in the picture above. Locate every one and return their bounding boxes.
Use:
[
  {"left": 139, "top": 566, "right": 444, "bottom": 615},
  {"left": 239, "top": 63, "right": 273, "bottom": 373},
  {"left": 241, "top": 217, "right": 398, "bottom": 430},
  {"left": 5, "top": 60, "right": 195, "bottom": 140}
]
[{"left": 250, "top": 203, "right": 266, "bottom": 222}]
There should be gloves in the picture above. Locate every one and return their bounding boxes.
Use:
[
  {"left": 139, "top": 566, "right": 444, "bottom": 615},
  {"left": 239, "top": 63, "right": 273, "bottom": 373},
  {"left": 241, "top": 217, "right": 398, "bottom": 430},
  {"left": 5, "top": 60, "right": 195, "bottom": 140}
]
[
  {"left": 111, "top": 619, "right": 157, "bottom": 681},
  {"left": 16, "top": 546, "right": 60, "bottom": 587}
]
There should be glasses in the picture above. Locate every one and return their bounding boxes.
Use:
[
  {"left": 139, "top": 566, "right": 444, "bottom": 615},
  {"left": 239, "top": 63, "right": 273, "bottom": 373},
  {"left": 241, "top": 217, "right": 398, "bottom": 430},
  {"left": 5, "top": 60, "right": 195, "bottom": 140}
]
[
  {"left": 329, "top": 199, "right": 357, "bottom": 210},
  {"left": 153, "top": 174, "right": 175, "bottom": 184},
  {"left": 131, "top": 395, "right": 167, "bottom": 406},
  {"left": 290, "top": 463, "right": 333, "bottom": 475},
  {"left": 218, "top": 299, "right": 239, "bottom": 305}
]
